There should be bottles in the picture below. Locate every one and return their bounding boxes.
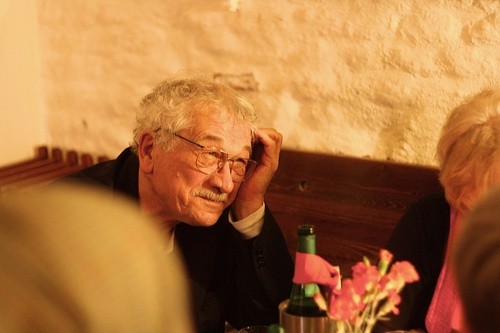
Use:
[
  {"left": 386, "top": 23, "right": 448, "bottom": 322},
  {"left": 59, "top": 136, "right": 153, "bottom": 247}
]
[{"left": 288, "top": 225, "right": 326, "bottom": 315}]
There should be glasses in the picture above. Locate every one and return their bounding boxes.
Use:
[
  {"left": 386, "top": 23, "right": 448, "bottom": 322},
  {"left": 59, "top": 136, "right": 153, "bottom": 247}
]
[{"left": 155, "top": 127, "right": 258, "bottom": 181}]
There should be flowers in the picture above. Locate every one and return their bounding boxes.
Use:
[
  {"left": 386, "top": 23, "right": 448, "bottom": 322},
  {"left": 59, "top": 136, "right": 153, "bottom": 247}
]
[{"left": 308, "top": 246, "right": 420, "bottom": 333}]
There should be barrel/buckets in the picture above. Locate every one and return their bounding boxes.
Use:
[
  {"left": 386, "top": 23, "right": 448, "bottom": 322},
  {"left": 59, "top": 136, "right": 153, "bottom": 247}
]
[{"left": 277, "top": 294, "right": 342, "bottom": 333}]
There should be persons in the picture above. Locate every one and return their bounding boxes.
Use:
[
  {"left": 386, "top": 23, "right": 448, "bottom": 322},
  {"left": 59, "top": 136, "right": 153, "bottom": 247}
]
[
  {"left": 0, "top": 181, "right": 193, "bottom": 333},
  {"left": 385, "top": 88, "right": 500, "bottom": 333},
  {"left": 456, "top": 191, "right": 500, "bottom": 333},
  {"left": 38, "top": 78, "right": 293, "bottom": 332}
]
